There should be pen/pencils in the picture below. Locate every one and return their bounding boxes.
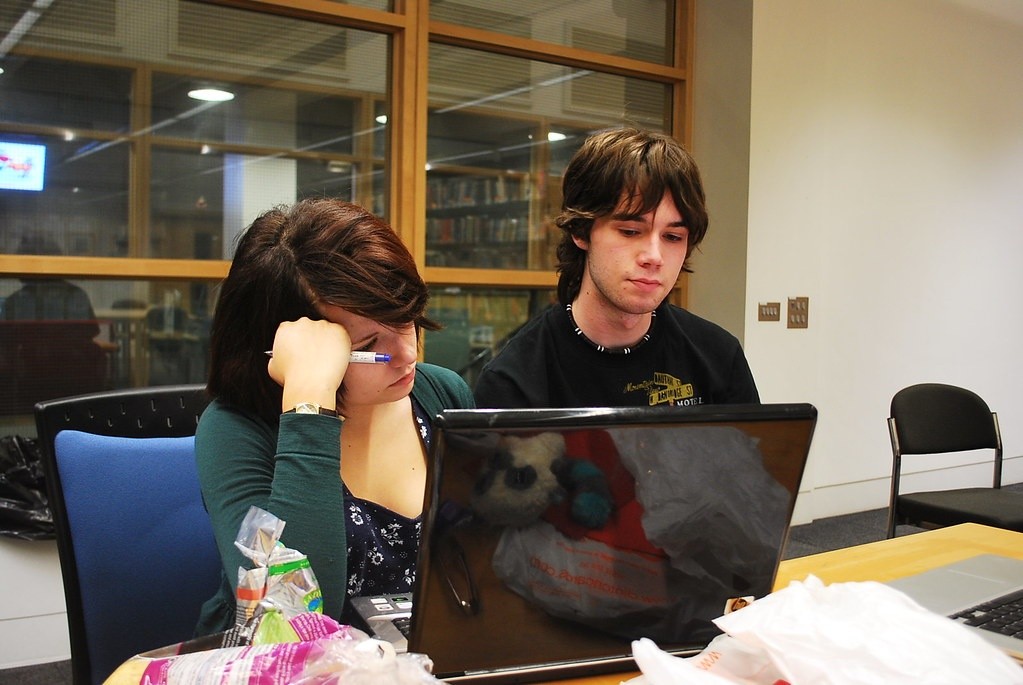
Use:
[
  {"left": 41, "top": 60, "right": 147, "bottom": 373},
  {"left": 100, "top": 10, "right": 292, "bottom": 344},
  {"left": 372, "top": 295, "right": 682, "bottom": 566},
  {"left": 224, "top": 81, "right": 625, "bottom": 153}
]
[{"left": 263, "top": 351, "right": 391, "bottom": 365}]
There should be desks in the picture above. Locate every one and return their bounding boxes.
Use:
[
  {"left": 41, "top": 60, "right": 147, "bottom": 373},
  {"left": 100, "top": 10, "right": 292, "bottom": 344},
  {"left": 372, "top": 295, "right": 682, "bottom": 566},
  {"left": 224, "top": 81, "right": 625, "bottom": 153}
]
[
  {"left": 94, "top": 307, "right": 149, "bottom": 378},
  {"left": 535, "top": 524, "right": 1023, "bottom": 685}
]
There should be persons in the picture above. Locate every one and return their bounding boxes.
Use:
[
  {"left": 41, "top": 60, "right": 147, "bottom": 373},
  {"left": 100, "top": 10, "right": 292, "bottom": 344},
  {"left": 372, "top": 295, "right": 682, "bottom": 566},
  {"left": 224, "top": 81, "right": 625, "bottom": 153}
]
[
  {"left": 0, "top": 236, "right": 101, "bottom": 340},
  {"left": 193, "top": 198, "right": 476, "bottom": 639},
  {"left": 473, "top": 127, "right": 761, "bottom": 409}
]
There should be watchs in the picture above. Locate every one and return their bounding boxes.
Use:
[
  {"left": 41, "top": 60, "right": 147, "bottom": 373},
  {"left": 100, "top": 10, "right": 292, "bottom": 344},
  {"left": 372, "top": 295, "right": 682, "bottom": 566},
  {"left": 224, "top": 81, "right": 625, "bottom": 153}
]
[{"left": 283, "top": 403, "right": 338, "bottom": 417}]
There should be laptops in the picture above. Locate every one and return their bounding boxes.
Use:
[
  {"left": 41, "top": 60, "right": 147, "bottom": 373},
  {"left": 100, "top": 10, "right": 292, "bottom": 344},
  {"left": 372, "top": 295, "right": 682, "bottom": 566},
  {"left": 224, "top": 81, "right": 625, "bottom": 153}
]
[
  {"left": 348, "top": 402, "right": 819, "bottom": 685},
  {"left": 881, "top": 553, "right": 1023, "bottom": 671}
]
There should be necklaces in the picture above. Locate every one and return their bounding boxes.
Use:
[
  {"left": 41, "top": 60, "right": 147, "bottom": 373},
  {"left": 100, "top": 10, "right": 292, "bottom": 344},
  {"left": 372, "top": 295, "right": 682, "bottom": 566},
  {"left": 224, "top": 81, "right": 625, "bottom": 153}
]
[{"left": 566, "top": 299, "right": 656, "bottom": 355}]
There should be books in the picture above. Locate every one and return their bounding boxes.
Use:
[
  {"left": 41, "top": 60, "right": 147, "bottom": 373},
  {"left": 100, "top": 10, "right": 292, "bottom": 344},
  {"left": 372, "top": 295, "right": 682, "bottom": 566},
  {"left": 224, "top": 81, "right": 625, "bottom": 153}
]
[{"left": 426, "top": 175, "right": 529, "bottom": 270}]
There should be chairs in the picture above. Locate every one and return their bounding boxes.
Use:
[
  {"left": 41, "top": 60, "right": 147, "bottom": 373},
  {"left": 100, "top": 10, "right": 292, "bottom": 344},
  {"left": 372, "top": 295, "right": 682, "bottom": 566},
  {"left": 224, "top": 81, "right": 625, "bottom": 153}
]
[
  {"left": 31, "top": 382, "right": 222, "bottom": 685},
  {"left": 886, "top": 382, "right": 1023, "bottom": 540},
  {"left": 106, "top": 298, "right": 207, "bottom": 389}
]
